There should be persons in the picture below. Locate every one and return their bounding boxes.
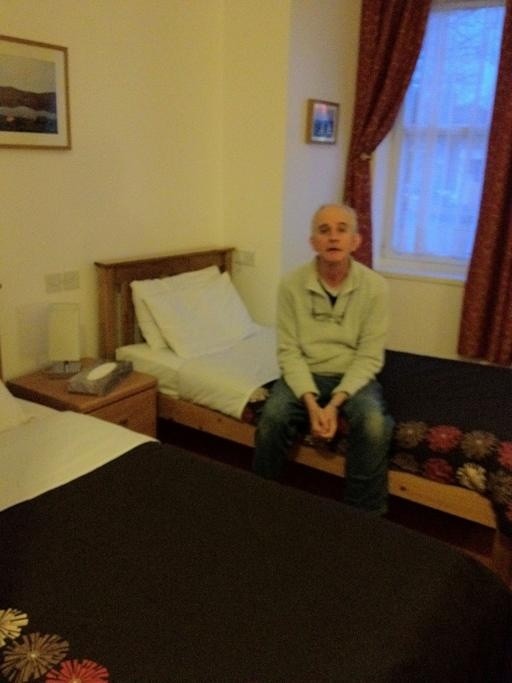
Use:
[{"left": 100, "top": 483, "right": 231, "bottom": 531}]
[{"left": 252, "top": 202, "right": 394, "bottom": 515}]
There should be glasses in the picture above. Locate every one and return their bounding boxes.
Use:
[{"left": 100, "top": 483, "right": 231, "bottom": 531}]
[{"left": 312, "top": 308, "right": 345, "bottom": 324}]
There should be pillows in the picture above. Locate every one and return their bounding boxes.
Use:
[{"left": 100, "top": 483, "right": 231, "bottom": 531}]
[{"left": 127, "top": 263, "right": 263, "bottom": 360}]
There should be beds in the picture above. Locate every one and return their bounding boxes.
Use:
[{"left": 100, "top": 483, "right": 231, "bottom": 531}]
[
  {"left": 94, "top": 245, "right": 510, "bottom": 559},
  {"left": 0, "top": 382, "right": 511, "bottom": 683}
]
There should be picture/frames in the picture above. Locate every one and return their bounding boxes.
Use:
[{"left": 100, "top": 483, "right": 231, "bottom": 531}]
[
  {"left": 1, "top": 32, "right": 73, "bottom": 150},
  {"left": 305, "top": 95, "right": 342, "bottom": 144}
]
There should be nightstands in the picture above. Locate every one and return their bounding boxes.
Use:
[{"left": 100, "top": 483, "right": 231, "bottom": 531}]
[{"left": 8, "top": 358, "right": 158, "bottom": 440}]
